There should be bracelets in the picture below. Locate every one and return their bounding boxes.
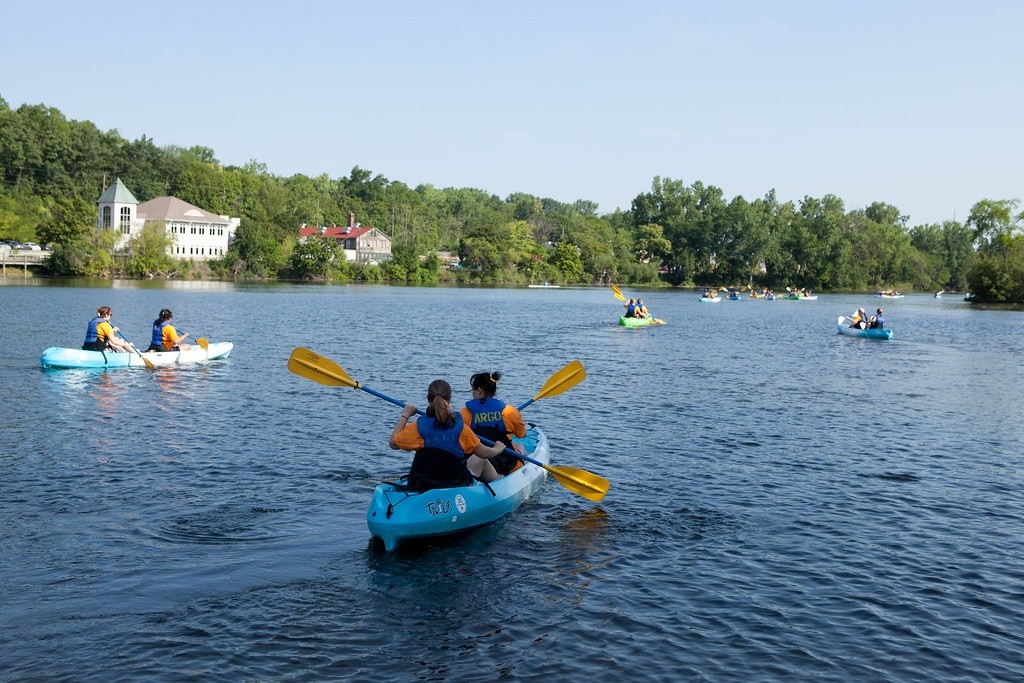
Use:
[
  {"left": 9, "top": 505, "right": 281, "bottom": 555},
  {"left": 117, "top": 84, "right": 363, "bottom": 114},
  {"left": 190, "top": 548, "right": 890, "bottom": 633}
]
[{"left": 400, "top": 414, "right": 409, "bottom": 420}]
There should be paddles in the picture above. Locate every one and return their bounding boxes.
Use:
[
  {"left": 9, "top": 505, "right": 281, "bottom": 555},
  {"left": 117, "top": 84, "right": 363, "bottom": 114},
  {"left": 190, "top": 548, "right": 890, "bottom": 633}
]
[
  {"left": 860, "top": 316, "right": 876, "bottom": 330},
  {"left": 786, "top": 287, "right": 791, "bottom": 291},
  {"left": 611, "top": 284, "right": 655, "bottom": 325},
  {"left": 756, "top": 294, "right": 783, "bottom": 298},
  {"left": 175, "top": 329, "right": 208, "bottom": 349},
  {"left": 837, "top": 311, "right": 858, "bottom": 325},
  {"left": 801, "top": 288, "right": 805, "bottom": 291},
  {"left": 748, "top": 285, "right": 752, "bottom": 291},
  {"left": 614, "top": 293, "right": 667, "bottom": 324},
  {"left": 517, "top": 360, "right": 587, "bottom": 411},
  {"left": 106, "top": 320, "right": 155, "bottom": 368},
  {"left": 286, "top": 346, "right": 611, "bottom": 503}
]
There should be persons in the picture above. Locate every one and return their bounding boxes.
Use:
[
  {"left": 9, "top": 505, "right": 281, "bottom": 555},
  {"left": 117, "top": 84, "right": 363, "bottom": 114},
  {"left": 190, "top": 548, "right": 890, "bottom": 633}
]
[
  {"left": 879, "top": 289, "right": 900, "bottom": 297},
  {"left": 703, "top": 284, "right": 811, "bottom": 300},
  {"left": 83, "top": 306, "right": 135, "bottom": 353},
  {"left": 853, "top": 307, "right": 884, "bottom": 329},
  {"left": 148, "top": 309, "right": 191, "bottom": 352},
  {"left": 389, "top": 371, "right": 527, "bottom": 494},
  {"left": 624, "top": 298, "right": 650, "bottom": 319}
]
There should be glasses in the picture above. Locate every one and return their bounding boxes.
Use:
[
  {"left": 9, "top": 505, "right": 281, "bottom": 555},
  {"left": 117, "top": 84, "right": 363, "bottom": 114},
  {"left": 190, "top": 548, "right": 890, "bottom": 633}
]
[{"left": 469, "top": 388, "right": 474, "bottom": 394}]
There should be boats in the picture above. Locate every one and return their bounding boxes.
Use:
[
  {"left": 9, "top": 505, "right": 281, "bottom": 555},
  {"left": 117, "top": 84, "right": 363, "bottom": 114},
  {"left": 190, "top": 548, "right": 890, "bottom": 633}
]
[
  {"left": 838, "top": 315, "right": 894, "bottom": 339},
  {"left": 699, "top": 297, "right": 722, "bottom": 302},
  {"left": 619, "top": 312, "right": 655, "bottom": 329},
  {"left": 42, "top": 339, "right": 237, "bottom": 373},
  {"left": 876, "top": 294, "right": 905, "bottom": 298},
  {"left": 748, "top": 293, "right": 817, "bottom": 300},
  {"left": 728, "top": 295, "right": 741, "bottom": 300},
  {"left": 366, "top": 422, "right": 553, "bottom": 553}
]
